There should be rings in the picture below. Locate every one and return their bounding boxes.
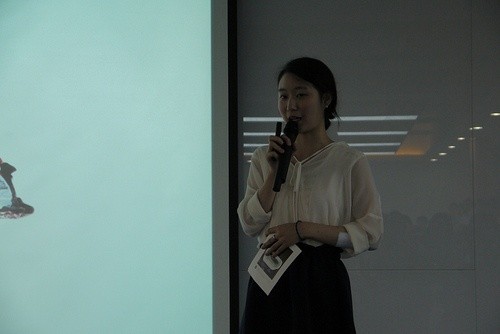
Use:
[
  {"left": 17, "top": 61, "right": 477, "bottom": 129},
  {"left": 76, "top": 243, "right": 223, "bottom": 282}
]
[{"left": 273, "top": 235, "right": 278, "bottom": 241}]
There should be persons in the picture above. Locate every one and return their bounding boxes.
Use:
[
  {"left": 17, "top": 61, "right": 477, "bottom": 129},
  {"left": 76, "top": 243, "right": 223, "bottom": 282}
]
[{"left": 237, "top": 57, "right": 384, "bottom": 334}]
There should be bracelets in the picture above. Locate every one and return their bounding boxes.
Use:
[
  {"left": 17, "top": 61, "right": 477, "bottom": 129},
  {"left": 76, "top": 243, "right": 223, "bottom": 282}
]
[{"left": 296, "top": 220, "right": 306, "bottom": 240}]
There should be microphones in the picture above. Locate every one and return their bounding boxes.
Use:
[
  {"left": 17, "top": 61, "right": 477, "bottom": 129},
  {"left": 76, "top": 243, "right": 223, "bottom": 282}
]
[{"left": 271, "top": 121, "right": 298, "bottom": 192}]
